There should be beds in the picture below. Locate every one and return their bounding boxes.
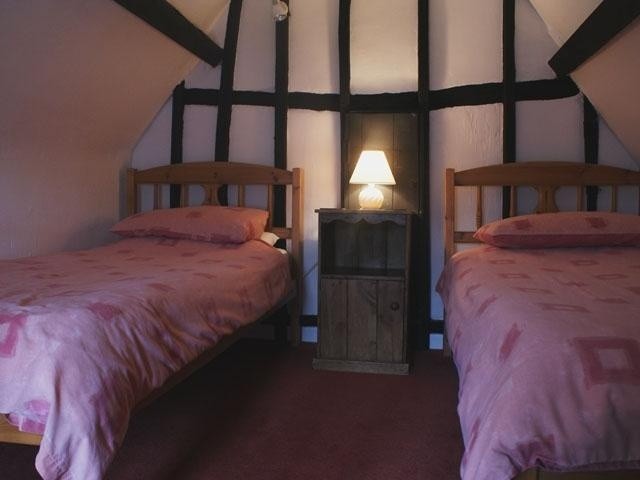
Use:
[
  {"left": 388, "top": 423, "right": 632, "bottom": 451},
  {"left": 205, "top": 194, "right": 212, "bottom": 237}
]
[
  {"left": 435, "top": 161, "right": 639, "bottom": 480},
  {"left": 1, "top": 162, "right": 303, "bottom": 480}
]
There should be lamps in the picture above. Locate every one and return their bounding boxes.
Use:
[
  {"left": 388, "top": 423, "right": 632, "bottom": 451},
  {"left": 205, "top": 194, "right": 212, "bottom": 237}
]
[{"left": 349, "top": 150, "right": 396, "bottom": 209}]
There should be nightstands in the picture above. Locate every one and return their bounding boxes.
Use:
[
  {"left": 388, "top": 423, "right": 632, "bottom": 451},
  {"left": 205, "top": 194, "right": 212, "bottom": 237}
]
[{"left": 313, "top": 208, "right": 422, "bottom": 376}]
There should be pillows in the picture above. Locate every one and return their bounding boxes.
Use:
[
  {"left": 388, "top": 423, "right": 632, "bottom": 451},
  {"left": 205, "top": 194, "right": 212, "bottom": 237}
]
[
  {"left": 108, "top": 207, "right": 269, "bottom": 245},
  {"left": 474, "top": 211, "right": 639, "bottom": 250}
]
